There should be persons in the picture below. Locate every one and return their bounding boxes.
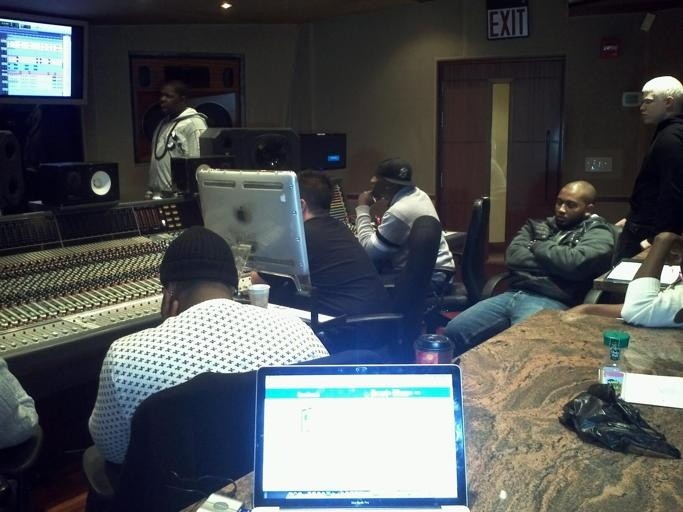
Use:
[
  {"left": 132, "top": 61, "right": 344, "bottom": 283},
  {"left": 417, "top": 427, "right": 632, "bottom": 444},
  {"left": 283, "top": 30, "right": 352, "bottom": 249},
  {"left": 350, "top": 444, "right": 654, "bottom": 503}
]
[
  {"left": 83, "top": 225, "right": 332, "bottom": 512},
  {"left": 0, "top": 356, "right": 42, "bottom": 494},
  {"left": 145, "top": 80, "right": 209, "bottom": 201},
  {"left": 352, "top": 157, "right": 456, "bottom": 300},
  {"left": 441, "top": 179, "right": 619, "bottom": 358},
  {"left": 620, "top": 231, "right": 683, "bottom": 327},
  {"left": 611, "top": 74, "right": 683, "bottom": 257},
  {"left": 249, "top": 169, "right": 397, "bottom": 354}
]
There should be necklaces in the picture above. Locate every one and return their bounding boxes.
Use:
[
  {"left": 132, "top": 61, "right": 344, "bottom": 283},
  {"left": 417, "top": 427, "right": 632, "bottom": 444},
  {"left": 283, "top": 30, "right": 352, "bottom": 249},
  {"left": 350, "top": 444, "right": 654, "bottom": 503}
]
[{"left": 153, "top": 119, "right": 181, "bottom": 160}]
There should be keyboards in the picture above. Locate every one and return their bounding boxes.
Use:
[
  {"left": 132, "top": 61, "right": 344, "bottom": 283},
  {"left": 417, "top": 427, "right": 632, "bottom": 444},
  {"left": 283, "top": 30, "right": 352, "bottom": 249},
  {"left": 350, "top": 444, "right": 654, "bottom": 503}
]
[{"left": 266, "top": 301, "right": 336, "bottom": 324}]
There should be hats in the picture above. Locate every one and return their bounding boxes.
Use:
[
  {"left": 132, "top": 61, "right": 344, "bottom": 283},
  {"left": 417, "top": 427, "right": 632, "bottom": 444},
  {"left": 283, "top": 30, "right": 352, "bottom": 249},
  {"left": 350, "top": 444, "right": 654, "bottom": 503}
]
[
  {"left": 160, "top": 228, "right": 238, "bottom": 287},
  {"left": 377, "top": 157, "right": 414, "bottom": 186}
]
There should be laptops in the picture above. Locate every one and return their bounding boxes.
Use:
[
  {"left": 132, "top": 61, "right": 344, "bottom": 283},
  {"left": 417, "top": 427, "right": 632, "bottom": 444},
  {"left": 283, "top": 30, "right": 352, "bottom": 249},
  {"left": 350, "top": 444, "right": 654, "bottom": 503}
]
[{"left": 253, "top": 365, "right": 470, "bottom": 511}]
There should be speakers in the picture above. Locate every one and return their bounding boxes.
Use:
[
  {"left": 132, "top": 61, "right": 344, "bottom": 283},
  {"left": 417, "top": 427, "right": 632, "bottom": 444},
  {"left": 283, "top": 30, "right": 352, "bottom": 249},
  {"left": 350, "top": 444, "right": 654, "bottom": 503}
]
[
  {"left": 170, "top": 154, "right": 233, "bottom": 195},
  {"left": 39, "top": 161, "right": 120, "bottom": 204},
  {"left": 199, "top": 128, "right": 295, "bottom": 170},
  {"left": 297, "top": 130, "right": 348, "bottom": 171}
]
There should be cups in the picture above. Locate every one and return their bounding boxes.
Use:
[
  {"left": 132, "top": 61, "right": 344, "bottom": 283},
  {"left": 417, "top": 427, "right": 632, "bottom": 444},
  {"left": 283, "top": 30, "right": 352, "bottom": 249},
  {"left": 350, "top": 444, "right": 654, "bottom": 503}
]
[
  {"left": 247, "top": 283, "right": 271, "bottom": 307},
  {"left": 412, "top": 332, "right": 454, "bottom": 365}
]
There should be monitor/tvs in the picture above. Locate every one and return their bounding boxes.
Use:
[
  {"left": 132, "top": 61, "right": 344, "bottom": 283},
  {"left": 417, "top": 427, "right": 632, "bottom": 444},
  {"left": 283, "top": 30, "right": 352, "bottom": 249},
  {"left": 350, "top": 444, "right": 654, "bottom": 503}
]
[
  {"left": 0, "top": 10, "right": 89, "bottom": 106},
  {"left": 196, "top": 166, "right": 313, "bottom": 297}
]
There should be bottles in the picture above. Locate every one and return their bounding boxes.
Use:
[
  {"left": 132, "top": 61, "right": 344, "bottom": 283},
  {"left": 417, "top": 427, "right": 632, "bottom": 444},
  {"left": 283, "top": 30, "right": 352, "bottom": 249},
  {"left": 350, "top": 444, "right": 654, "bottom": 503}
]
[{"left": 599, "top": 329, "right": 629, "bottom": 400}]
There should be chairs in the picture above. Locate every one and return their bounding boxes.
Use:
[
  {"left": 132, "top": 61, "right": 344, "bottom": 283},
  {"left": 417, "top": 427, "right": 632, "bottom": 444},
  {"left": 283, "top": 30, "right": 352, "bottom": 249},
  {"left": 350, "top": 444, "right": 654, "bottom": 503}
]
[
  {"left": 480, "top": 221, "right": 620, "bottom": 303},
  {"left": 83, "top": 350, "right": 413, "bottom": 512},
  {"left": 345, "top": 195, "right": 490, "bottom": 364}
]
